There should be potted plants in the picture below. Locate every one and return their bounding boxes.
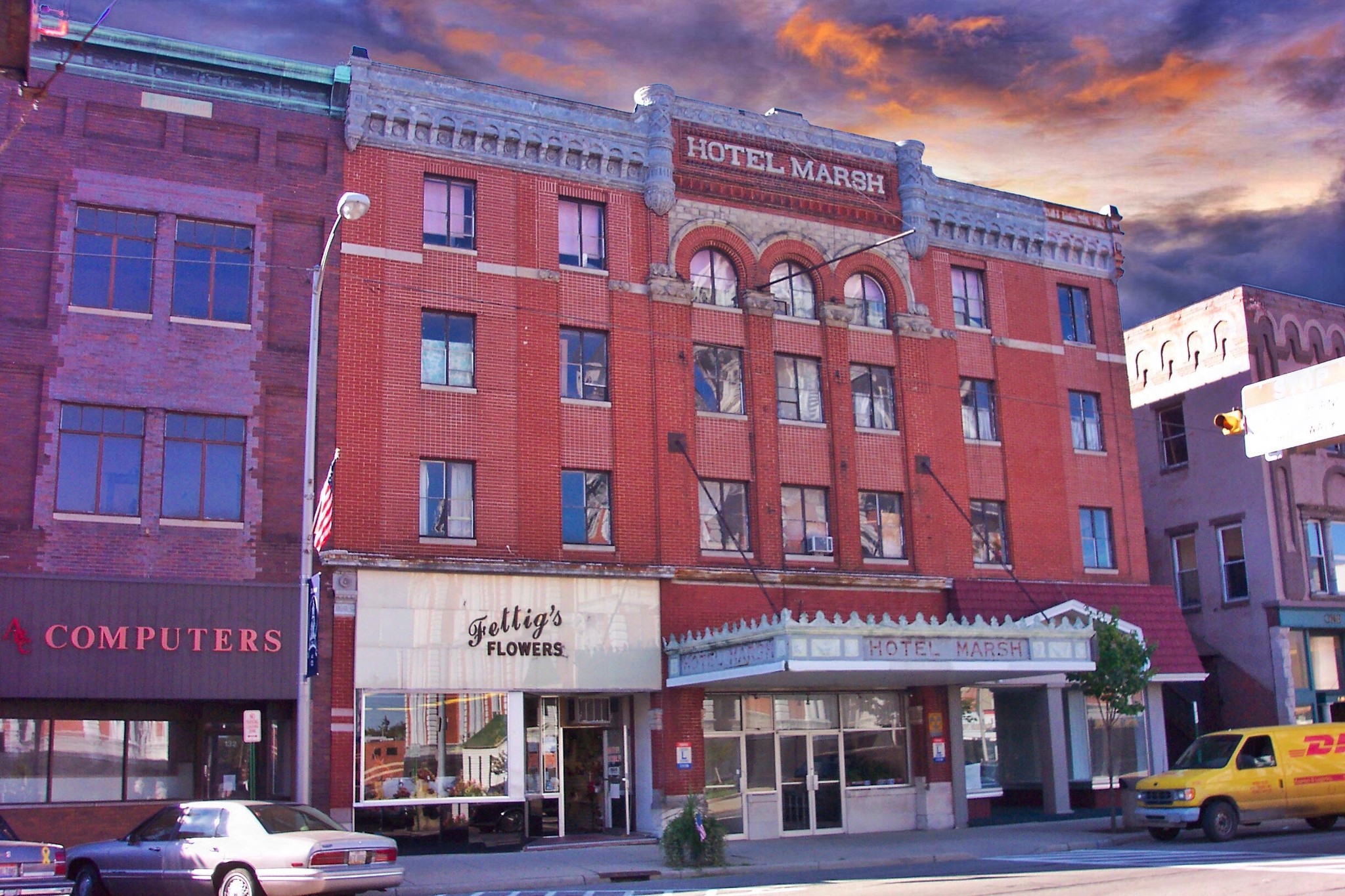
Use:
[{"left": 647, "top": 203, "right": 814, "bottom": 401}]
[{"left": 655, "top": 778, "right": 729, "bottom": 876}]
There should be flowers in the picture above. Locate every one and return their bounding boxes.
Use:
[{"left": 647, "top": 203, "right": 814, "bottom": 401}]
[{"left": 443, "top": 813, "right": 469, "bottom": 829}]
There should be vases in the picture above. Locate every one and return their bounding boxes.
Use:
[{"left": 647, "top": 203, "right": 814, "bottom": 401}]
[{"left": 438, "top": 822, "right": 469, "bottom": 852}]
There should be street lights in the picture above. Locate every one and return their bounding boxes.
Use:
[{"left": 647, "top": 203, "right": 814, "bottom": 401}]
[{"left": 295, "top": 190, "right": 373, "bottom": 831}]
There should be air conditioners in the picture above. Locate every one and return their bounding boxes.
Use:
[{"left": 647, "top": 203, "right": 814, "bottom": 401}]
[{"left": 807, "top": 536, "right": 833, "bottom": 553}]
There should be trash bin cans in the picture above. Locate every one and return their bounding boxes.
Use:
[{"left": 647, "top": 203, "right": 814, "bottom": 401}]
[{"left": 1117, "top": 776, "right": 1148, "bottom": 830}]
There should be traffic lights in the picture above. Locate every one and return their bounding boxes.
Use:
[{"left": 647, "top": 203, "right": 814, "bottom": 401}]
[{"left": 1214, "top": 408, "right": 1242, "bottom": 436}]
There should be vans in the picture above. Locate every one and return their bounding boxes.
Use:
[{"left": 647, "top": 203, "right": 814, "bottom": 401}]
[{"left": 1134, "top": 721, "right": 1345, "bottom": 843}]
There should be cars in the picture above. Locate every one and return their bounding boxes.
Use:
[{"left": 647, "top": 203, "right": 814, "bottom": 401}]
[
  {"left": 63, "top": 799, "right": 406, "bottom": 896},
  {"left": 0, "top": 813, "right": 77, "bottom": 896}
]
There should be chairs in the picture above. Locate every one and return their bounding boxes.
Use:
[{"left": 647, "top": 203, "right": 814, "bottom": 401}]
[{"left": 1241, "top": 739, "right": 1263, "bottom": 768}]
